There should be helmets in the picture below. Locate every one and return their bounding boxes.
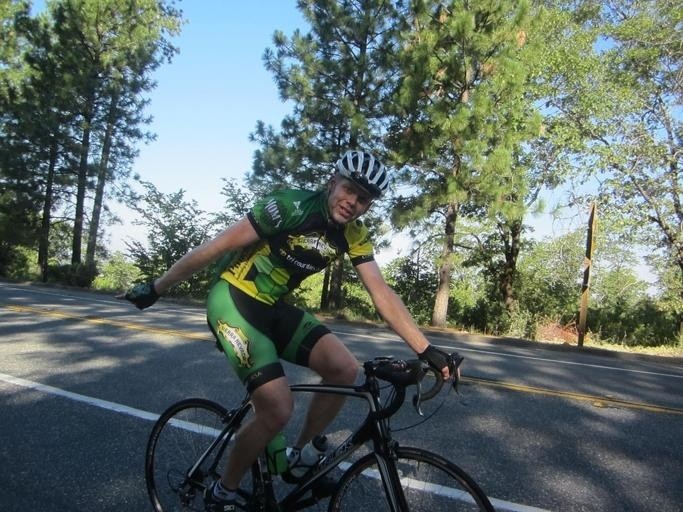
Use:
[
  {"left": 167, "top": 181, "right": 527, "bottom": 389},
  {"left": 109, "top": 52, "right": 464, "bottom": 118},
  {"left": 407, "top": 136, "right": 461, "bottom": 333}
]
[{"left": 335, "top": 150, "right": 389, "bottom": 198}]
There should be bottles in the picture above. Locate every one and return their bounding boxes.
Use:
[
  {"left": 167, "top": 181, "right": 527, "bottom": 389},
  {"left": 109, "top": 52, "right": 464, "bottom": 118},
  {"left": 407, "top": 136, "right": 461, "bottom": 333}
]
[
  {"left": 301, "top": 434, "right": 328, "bottom": 467},
  {"left": 267, "top": 431, "right": 288, "bottom": 476}
]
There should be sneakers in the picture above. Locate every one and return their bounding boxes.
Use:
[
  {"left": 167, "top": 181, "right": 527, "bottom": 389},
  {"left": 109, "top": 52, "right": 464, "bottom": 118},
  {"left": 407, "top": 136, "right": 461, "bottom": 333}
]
[
  {"left": 202, "top": 481, "right": 236, "bottom": 511},
  {"left": 281, "top": 447, "right": 346, "bottom": 489}
]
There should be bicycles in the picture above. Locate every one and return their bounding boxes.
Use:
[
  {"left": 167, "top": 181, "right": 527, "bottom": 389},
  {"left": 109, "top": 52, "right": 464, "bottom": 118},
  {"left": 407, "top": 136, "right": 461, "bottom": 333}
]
[{"left": 146, "top": 352, "right": 497, "bottom": 512}]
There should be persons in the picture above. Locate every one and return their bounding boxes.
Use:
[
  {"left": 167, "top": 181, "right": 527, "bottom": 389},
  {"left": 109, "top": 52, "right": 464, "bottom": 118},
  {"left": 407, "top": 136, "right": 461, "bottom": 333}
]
[{"left": 115, "top": 149, "right": 461, "bottom": 512}]
